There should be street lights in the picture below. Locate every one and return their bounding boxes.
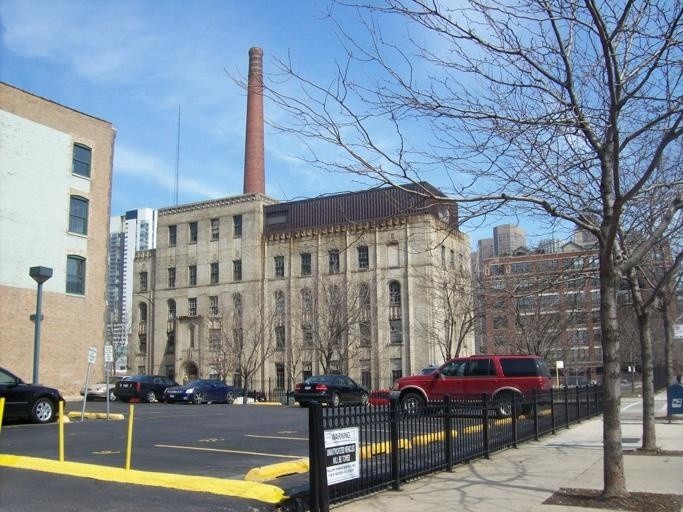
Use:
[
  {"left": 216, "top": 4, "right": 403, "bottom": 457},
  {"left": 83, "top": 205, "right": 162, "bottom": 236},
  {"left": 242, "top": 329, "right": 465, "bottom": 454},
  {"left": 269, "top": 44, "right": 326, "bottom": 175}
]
[{"left": 28, "top": 264, "right": 52, "bottom": 383}]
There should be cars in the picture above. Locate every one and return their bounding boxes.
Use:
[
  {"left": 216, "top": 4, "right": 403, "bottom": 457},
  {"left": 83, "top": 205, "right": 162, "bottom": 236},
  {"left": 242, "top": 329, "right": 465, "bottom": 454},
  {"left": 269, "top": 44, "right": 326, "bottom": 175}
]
[
  {"left": 367, "top": 389, "right": 390, "bottom": 405},
  {"left": 115, "top": 373, "right": 181, "bottom": 403},
  {"left": 294, "top": 375, "right": 368, "bottom": 407},
  {"left": 78, "top": 375, "right": 130, "bottom": 402},
  {"left": 162, "top": 380, "right": 239, "bottom": 404},
  {"left": 0, "top": 367, "right": 64, "bottom": 427}
]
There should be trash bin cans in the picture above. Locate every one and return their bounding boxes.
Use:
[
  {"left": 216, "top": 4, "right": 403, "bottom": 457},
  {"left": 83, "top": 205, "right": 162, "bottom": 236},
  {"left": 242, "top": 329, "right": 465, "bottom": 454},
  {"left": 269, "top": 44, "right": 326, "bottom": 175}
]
[
  {"left": 667, "top": 383, "right": 683, "bottom": 418},
  {"left": 286, "top": 391, "right": 294, "bottom": 405}
]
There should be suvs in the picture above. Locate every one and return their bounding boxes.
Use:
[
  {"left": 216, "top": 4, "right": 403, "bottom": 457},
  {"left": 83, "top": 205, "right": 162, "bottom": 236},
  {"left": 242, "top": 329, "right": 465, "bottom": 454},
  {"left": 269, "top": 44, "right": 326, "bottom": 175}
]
[{"left": 390, "top": 355, "right": 553, "bottom": 416}]
